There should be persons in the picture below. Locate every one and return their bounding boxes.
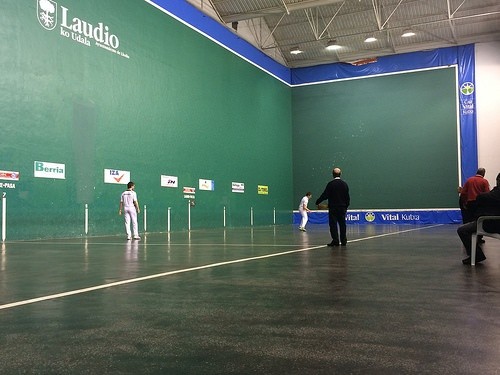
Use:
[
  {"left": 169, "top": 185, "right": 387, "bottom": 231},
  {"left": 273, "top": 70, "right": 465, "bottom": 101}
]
[
  {"left": 299, "top": 192, "right": 313, "bottom": 231},
  {"left": 119, "top": 181, "right": 141, "bottom": 239},
  {"left": 457, "top": 168, "right": 500, "bottom": 265},
  {"left": 315, "top": 168, "right": 350, "bottom": 246}
]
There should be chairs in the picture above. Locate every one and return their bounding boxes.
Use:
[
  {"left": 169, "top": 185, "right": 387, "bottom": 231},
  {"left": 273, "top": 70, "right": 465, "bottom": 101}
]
[{"left": 471, "top": 215, "right": 500, "bottom": 266}]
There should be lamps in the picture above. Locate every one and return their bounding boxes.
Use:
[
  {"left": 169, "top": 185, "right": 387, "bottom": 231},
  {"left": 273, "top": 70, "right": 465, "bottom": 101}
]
[
  {"left": 401, "top": 29, "right": 415, "bottom": 37},
  {"left": 326, "top": 41, "right": 342, "bottom": 50},
  {"left": 364, "top": 37, "right": 377, "bottom": 43},
  {"left": 290, "top": 47, "right": 304, "bottom": 54}
]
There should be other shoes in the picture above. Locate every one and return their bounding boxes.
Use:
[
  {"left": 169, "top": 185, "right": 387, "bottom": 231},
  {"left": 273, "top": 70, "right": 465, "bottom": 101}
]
[
  {"left": 127, "top": 237, "right": 132, "bottom": 240},
  {"left": 341, "top": 243, "right": 346, "bottom": 246},
  {"left": 134, "top": 236, "right": 140, "bottom": 239},
  {"left": 462, "top": 253, "right": 486, "bottom": 263},
  {"left": 327, "top": 242, "right": 339, "bottom": 246}
]
